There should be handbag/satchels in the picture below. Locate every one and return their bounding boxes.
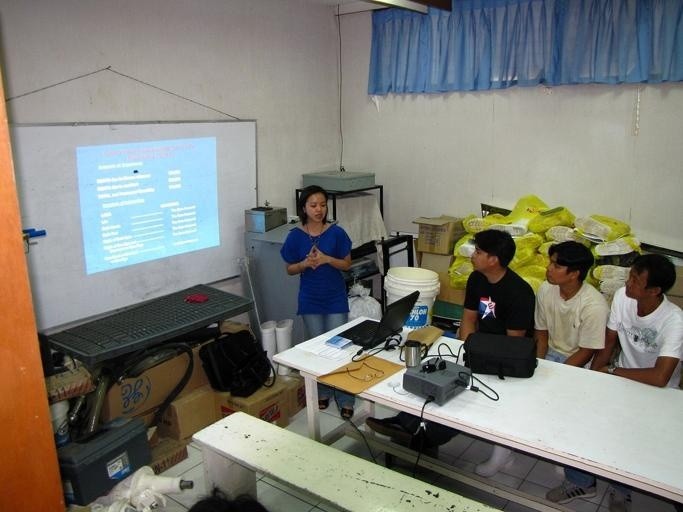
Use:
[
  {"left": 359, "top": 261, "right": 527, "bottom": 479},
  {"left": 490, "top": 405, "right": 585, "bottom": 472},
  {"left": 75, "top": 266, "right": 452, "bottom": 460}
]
[
  {"left": 463, "top": 327, "right": 537, "bottom": 379},
  {"left": 199, "top": 330, "right": 275, "bottom": 397}
]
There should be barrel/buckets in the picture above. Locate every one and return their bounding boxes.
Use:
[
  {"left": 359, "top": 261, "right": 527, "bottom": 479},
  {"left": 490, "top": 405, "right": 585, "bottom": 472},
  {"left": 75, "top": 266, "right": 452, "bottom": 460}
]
[
  {"left": 48, "top": 400, "right": 71, "bottom": 449},
  {"left": 382, "top": 264, "right": 441, "bottom": 335}
]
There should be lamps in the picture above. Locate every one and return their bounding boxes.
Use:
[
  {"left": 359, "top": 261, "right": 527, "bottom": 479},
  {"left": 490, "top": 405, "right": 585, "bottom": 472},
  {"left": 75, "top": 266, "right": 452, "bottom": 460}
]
[{"left": 360, "top": 0, "right": 452, "bottom": 15}]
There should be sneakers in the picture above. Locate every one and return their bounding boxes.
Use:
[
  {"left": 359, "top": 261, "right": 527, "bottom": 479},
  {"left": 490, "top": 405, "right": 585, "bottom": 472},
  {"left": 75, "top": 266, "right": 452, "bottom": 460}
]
[
  {"left": 391, "top": 426, "right": 438, "bottom": 459},
  {"left": 366, "top": 412, "right": 421, "bottom": 436},
  {"left": 547, "top": 478, "right": 597, "bottom": 504},
  {"left": 609, "top": 486, "right": 632, "bottom": 512}
]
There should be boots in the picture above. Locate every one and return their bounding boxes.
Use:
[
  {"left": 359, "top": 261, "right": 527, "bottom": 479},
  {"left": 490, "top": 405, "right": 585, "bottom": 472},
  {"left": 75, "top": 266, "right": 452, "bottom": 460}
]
[
  {"left": 474, "top": 445, "right": 505, "bottom": 478},
  {"left": 448, "top": 194, "right": 643, "bottom": 309}
]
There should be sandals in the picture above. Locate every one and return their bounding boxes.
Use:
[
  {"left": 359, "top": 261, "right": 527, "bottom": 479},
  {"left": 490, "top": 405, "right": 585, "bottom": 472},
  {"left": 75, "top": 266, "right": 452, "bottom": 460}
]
[
  {"left": 319, "top": 397, "right": 329, "bottom": 409},
  {"left": 341, "top": 406, "right": 353, "bottom": 418}
]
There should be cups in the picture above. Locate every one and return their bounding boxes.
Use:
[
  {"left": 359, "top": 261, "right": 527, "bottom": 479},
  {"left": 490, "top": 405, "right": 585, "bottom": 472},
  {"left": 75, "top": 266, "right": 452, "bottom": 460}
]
[{"left": 405, "top": 340, "right": 428, "bottom": 368}]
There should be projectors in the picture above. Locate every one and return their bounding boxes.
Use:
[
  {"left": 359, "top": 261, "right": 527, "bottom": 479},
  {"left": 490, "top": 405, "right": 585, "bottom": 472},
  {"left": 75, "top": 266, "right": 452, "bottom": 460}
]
[{"left": 402, "top": 356, "right": 472, "bottom": 407}]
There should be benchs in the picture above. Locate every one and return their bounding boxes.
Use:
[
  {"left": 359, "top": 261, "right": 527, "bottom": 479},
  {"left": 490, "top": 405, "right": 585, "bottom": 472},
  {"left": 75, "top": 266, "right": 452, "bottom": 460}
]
[{"left": 192, "top": 411, "right": 507, "bottom": 512}]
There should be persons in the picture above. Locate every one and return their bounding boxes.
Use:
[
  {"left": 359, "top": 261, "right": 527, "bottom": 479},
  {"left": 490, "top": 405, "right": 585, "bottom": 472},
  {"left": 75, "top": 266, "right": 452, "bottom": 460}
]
[
  {"left": 280, "top": 185, "right": 355, "bottom": 421},
  {"left": 547, "top": 252, "right": 683, "bottom": 512},
  {"left": 475, "top": 240, "right": 610, "bottom": 479},
  {"left": 187, "top": 485, "right": 267, "bottom": 512},
  {"left": 366, "top": 228, "right": 536, "bottom": 449}
]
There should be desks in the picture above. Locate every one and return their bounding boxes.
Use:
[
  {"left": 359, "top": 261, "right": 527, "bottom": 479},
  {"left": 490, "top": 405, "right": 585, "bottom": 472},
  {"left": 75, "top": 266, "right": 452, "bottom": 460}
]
[{"left": 272, "top": 316, "right": 683, "bottom": 512}]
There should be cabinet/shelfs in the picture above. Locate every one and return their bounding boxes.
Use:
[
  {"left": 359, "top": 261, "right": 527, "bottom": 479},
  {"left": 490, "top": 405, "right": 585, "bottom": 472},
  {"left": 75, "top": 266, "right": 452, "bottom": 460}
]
[{"left": 295, "top": 184, "right": 385, "bottom": 318}]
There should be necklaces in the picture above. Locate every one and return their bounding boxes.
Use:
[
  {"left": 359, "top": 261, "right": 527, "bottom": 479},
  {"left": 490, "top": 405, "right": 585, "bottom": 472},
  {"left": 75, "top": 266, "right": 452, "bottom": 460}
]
[{"left": 305, "top": 222, "right": 324, "bottom": 249}]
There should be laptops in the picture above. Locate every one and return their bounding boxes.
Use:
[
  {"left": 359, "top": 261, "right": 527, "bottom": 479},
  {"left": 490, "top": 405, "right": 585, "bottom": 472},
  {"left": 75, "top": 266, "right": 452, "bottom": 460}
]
[{"left": 336, "top": 290, "right": 420, "bottom": 348}]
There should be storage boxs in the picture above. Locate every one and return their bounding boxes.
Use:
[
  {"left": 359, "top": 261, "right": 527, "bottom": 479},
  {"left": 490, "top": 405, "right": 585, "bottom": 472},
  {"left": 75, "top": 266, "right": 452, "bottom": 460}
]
[
  {"left": 271, "top": 374, "right": 305, "bottom": 427},
  {"left": 148, "top": 431, "right": 190, "bottom": 477},
  {"left": 667, "top": 296, "right": 683, "bottom": 311},
  {"left": 665, "top": 266, "right": 683, "bottom": 297},
  {"left": 214, "top": 380, "right": 291, "bottom": 431},
  {"left": 154, "top": 385, "right": 225, "bottom": 443},
  {"left": 58, "top": 415, "right": 149, "bottom": 506},
  {"left": 412, "top": 215, "right": 467, "bottom": 321},
  {"left": 83, "top": 320, "right": 257, "bottom": 422}
]
